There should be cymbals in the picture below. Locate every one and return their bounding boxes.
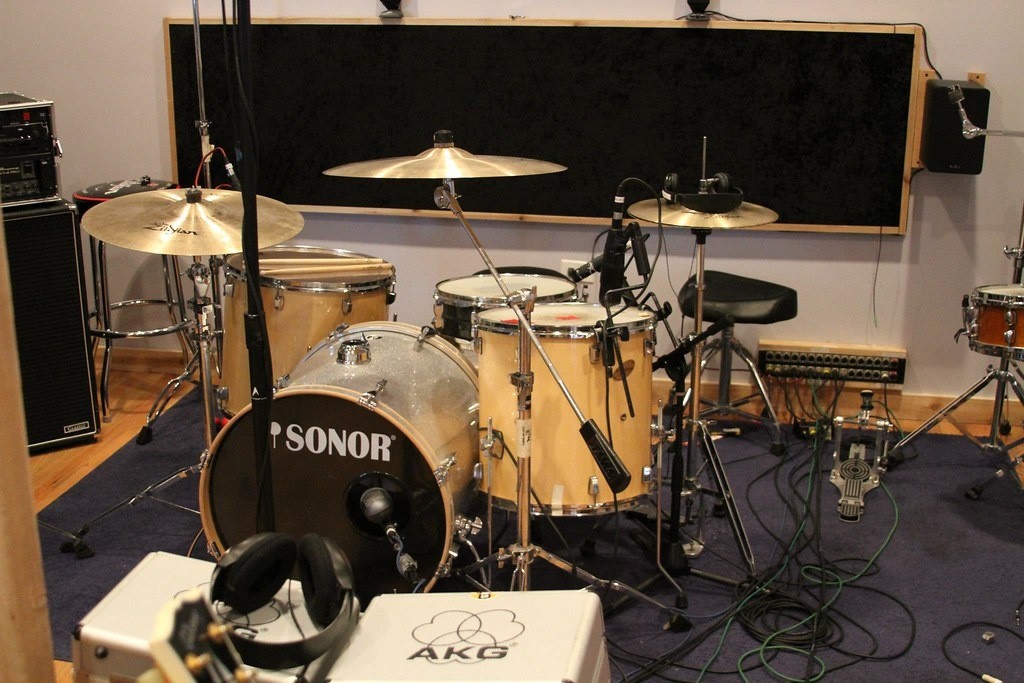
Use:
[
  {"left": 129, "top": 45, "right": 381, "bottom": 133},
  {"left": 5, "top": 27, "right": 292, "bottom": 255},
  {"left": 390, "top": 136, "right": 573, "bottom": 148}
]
[
  {"left": 321, "top": 146, "right": 569, "bottom": 181},
  {"left": 626, "top": 197, "right": 781, "bottom": 232},
  {"left": 80, "top": 186, "right": 306, "bottom": 257}
]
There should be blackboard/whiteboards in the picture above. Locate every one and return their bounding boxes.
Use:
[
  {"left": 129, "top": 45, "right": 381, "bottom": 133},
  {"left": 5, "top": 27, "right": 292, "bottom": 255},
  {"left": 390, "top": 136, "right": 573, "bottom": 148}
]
[{"left": 164, "top": 17, "right": 925, "bottom": 235}]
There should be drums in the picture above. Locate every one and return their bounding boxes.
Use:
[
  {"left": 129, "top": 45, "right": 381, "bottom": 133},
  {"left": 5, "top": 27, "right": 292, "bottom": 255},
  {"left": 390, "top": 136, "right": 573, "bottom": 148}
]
[
  {"left": 196, "top": 318, "right": 480, "bottom": 613},
  {"left": 430, "top": 272, "right": 579, "bottom": 342},
  {"left": 953, "top": 283, "right": 1024, "bottom": 363},
  {"left": 475, "top": 300, "right": 657, "bottom": 517},
  {"left": 215, "top": 244, "right": 398, "bottom": 419}
]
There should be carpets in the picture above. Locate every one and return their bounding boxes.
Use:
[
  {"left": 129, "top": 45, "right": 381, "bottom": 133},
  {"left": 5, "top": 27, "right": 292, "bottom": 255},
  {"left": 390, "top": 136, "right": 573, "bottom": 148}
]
[{"left": 35, "top": 386, "right": 1024, "bottom": 683}]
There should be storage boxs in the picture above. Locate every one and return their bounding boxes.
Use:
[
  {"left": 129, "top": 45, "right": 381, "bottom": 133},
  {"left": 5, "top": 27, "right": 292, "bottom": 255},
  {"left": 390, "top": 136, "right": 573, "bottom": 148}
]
[{"left": 71, "top": 550, "right": 611, "bottom": 683}]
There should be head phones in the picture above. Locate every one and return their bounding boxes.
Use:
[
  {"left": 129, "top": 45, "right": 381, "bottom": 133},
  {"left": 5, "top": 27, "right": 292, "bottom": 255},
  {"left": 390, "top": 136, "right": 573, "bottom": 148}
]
[
  {"left": 198, "top": 530, "right": 359, "bottom": 669},
  {"left": 662, "top": 173, "right": 744, "bottom": 214}
]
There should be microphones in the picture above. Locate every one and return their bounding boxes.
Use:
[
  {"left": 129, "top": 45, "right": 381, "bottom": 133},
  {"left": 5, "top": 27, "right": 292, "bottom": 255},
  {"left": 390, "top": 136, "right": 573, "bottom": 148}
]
[
  {"left": 361, "top": 487, "right": 394, "bottom": 524},
  {"left": 599, "top": 185, "right": 626, "bottom": 309},
  {"left": 222, "top": 157, "right": 241, "bottom": 191},
  {"left": 570, "top": 255, "right": 605, "bottom": 283}
]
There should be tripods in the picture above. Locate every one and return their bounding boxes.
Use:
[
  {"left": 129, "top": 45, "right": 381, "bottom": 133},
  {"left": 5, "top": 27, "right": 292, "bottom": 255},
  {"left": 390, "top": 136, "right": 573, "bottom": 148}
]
[
  {"left": 435, "top": 178, "right": 761, "bottom": 636},
  {"left": 886, "top": 357, "right": 1024, "bottom": 472}
]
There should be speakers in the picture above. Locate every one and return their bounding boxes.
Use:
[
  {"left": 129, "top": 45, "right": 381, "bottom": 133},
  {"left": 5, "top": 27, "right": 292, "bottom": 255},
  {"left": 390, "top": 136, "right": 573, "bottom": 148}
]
[
  {"left": 3, "top": 198, "right": 101, "bottom": 453},
  {"left": 920, "top": 79, "right": 991, "bottom": 175}
]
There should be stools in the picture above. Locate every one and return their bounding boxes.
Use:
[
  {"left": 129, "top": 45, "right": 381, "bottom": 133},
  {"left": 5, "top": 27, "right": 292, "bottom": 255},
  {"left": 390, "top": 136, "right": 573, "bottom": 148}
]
[
  {"left": 672, "top": 270, "right": 797, "bottom": 455},
  {"left": 73, "top": 180, "right": 198, "bottom": 423}
]
